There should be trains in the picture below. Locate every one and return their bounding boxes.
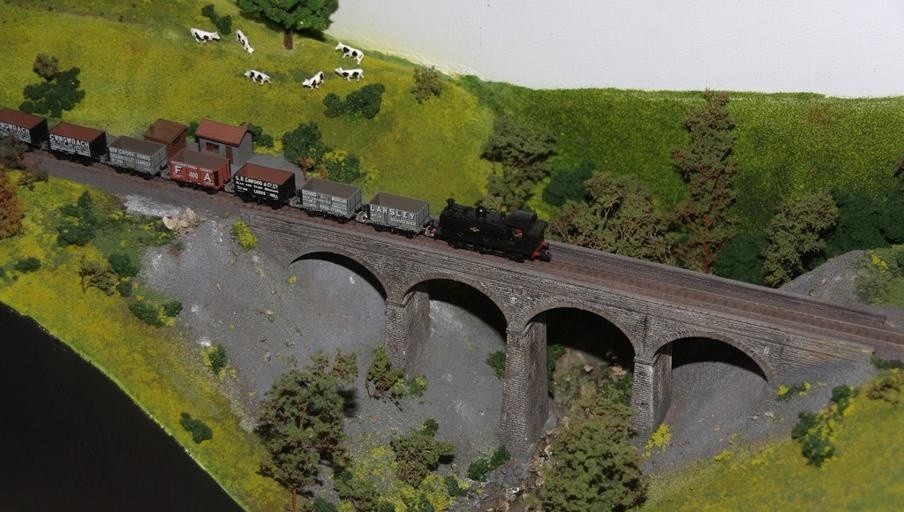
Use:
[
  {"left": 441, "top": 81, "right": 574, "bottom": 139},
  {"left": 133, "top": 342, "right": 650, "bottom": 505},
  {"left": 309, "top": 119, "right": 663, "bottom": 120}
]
[{"left": 0, "top": 108, "right": 552, "bottom": 263}]
